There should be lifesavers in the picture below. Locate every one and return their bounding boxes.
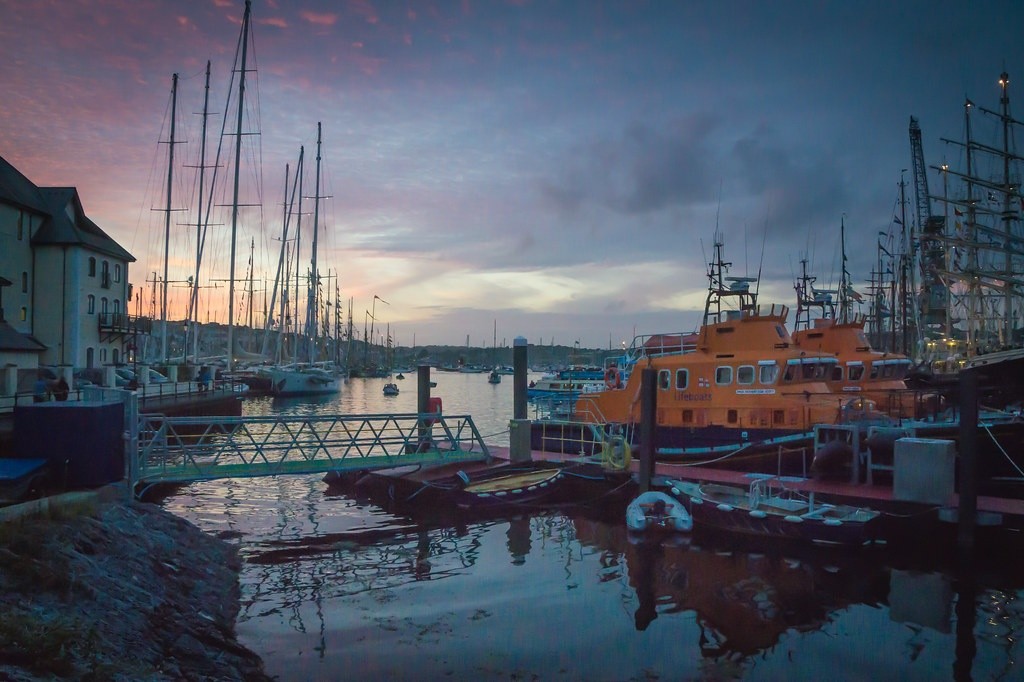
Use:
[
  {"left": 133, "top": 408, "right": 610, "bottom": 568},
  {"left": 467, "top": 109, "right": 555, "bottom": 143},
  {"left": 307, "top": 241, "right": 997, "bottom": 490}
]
[
  {"left": 605, "top": 436, "right": 632, "bottom": 472},
  {"left": 604, "top": 367, "right": 620, "bottom": 389}
]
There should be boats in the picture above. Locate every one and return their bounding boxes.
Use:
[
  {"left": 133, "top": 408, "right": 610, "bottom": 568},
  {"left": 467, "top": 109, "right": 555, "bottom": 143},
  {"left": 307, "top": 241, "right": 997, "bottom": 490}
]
[
  {"left": 625, "top": 490, "right": 694, "bottom": 534},
  {"left": 450, "top": 463, "right": 567, "bottom": 511},
  {"left": 421, "top": 460, "right": 518, "bottom": 506},
  {"left": 654, "top": 472, "right": 887, "bottom": 546}
]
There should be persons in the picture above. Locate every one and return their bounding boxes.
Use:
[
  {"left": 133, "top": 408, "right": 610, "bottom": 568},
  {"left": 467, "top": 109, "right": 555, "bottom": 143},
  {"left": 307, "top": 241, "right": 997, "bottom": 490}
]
[
  {"left": 32, "top": 376, "right": 69, "bottom": 402},
  {"left": 196, "top": 361, "right": 223, "bottom": 394}
]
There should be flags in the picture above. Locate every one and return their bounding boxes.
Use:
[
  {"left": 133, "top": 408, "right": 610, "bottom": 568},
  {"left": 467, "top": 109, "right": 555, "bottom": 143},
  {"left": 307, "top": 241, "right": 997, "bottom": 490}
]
[{"left": 847, "top": 288, "right": 864, "bottom": 304}]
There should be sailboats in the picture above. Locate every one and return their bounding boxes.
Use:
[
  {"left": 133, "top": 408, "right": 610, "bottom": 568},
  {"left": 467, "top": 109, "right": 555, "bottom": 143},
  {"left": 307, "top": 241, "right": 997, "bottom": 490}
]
[
  {"left": 0, "top": 2, "right": 428, "bottom": 506},
  {"left": 527, "top": 69, "right": 1022, "bottom": 500},
  {"left": 439, "top": 319, "right": 515, "bottom": 384}
]
